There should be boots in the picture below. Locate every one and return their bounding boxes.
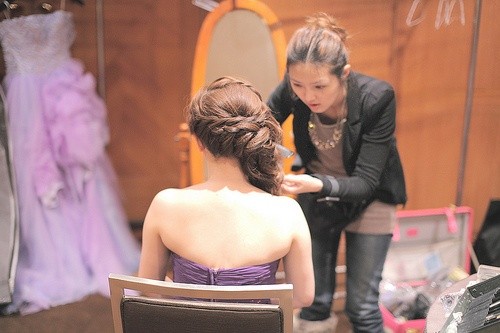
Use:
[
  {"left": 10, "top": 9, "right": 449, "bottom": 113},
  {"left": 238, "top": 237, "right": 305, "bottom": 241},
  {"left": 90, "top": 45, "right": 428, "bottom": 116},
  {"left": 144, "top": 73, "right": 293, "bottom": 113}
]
[{"left": 293, "top": 309, "right": 338, "bottom": 332}]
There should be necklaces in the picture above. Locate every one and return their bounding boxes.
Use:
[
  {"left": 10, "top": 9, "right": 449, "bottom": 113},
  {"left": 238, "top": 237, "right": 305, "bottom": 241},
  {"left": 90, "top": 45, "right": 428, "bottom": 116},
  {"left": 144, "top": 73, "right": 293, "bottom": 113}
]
[{"left": 308, "top": 96, "right": 346, "bottom": 152}]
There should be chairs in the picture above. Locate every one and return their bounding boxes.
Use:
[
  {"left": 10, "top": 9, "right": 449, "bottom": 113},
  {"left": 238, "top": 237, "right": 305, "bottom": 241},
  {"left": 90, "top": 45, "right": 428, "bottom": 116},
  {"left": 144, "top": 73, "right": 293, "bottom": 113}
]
[{"left": 108, "top": 270, "right": 294, "bottom": 333}]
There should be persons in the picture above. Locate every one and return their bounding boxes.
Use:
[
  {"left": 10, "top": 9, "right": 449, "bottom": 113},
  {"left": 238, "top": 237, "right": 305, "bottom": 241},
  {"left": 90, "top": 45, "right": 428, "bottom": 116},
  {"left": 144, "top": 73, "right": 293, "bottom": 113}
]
[
  {"left": 266, "top": 8, "right": 409, "bottom": 333},
  {"left": 134, "top": 72, "right": 318, "bottom": 312}
]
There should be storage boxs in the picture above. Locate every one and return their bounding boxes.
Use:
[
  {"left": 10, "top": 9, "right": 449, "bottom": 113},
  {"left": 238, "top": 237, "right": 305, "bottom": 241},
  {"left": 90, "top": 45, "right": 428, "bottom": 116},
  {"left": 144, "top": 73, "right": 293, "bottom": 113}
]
[{"left": 376, "top": 203, "right": 479, "bottom": 333}]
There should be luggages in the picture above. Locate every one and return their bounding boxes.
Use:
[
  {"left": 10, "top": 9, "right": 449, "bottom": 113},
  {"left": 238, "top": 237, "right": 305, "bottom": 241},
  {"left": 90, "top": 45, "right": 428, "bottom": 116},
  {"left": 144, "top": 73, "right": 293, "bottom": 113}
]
[{"left": 379, "top": 206, "right": 476, "bottom": 332}]
